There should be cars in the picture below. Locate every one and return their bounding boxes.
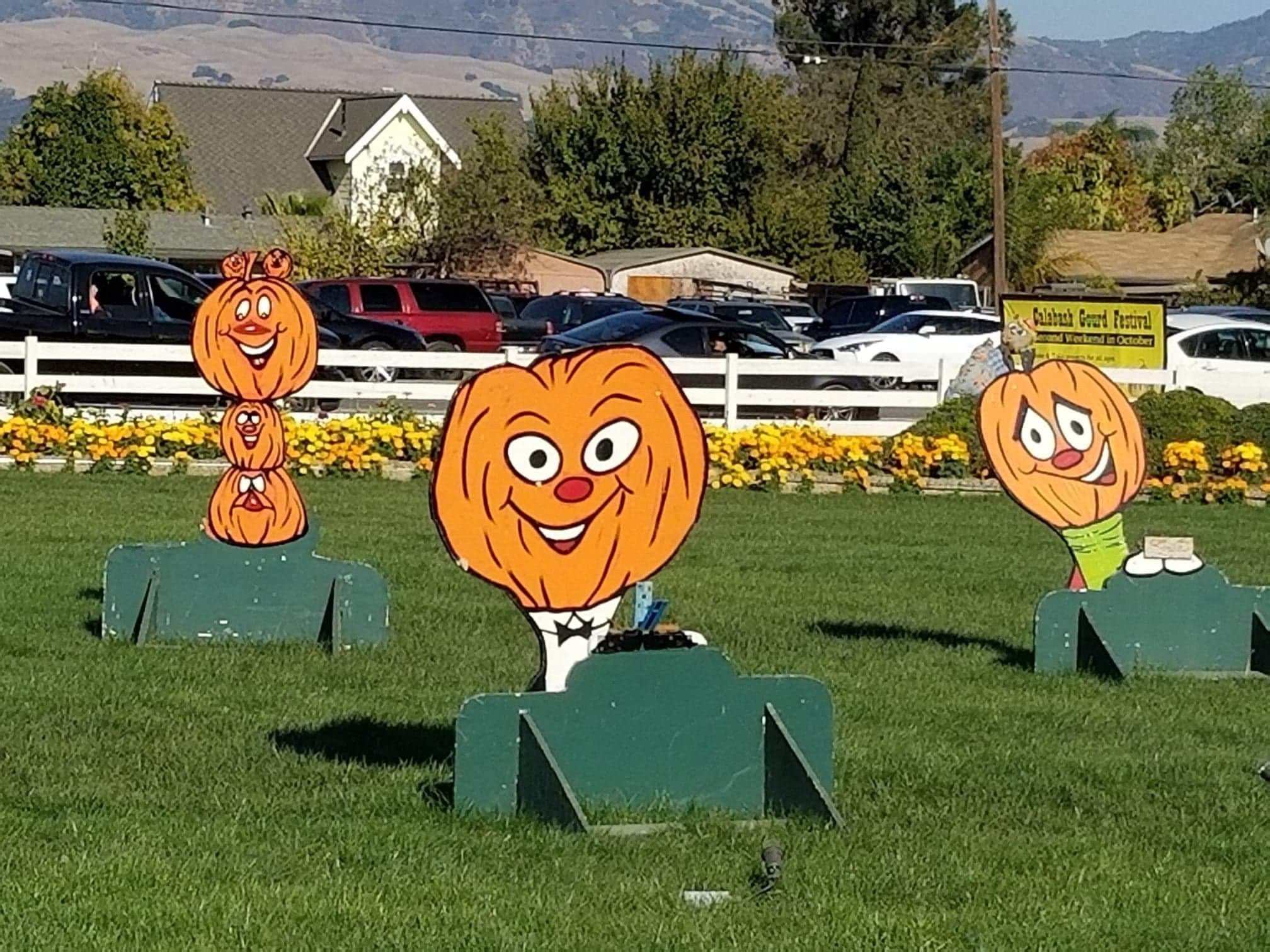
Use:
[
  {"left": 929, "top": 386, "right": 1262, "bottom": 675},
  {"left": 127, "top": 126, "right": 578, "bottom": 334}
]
[
  {"left": 1166, "top": 306, "right": 1270, "bottom": 326},
  {"left": 1162, "top": 314, "right": 1270, "bottom": 408},
  {"left": 0, "top": 244, "right": 1004, "bottom": 424}
]
